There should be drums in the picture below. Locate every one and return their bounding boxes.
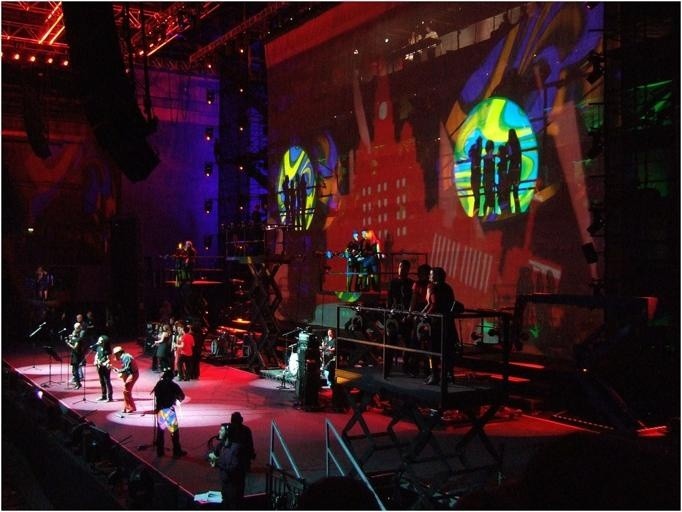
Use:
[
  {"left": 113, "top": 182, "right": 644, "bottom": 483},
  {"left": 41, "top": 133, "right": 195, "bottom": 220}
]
[{"left": 288, "top": 353, "right": 299, "bottom": 376}]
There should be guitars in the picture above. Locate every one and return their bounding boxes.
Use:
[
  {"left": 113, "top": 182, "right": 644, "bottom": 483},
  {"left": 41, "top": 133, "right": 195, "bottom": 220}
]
[{"left": 107, "top": 364, "right": 127, "bottom": 378}]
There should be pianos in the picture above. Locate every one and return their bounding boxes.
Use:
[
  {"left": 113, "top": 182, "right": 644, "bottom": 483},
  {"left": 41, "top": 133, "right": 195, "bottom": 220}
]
[{"left": 216, "top": 326, "right": 249, "bottom": 338}]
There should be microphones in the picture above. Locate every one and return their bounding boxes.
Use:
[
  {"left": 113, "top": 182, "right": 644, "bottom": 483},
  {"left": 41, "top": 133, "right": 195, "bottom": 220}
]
[
  {"left": 90, "top": 342, "right": 99, "bottom": 348},
  {"left": 159, "top": 371, "right": 164, "bottom": 378},
  {"left": 39, "top": 322, "right": 47, "bottom": 327},
  {"left": 58, "top": 328, "right": 67, "bottom": 334},
  {"left": 297, "top": 326, "right": 304, "bottom": 331},
  {"left": 65, "top": 337, "right": 68, "bottom": 343}
]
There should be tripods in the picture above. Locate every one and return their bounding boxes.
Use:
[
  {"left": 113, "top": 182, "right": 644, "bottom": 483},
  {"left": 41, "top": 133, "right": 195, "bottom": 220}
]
[
  {"left": 55, "top": 336, "right": 73, "bottom": 384},
  {"left": 23, "top": 327, "right": 42, "bottom": 371},
  {"left": 72, "top": 349, "right": 97, "bottom": 406},
  {"left": 138, "top": 378, "right": 171, "bottom": 452},
  {"left": 40, "top": 349, "right": 62, "bottom": 387}
]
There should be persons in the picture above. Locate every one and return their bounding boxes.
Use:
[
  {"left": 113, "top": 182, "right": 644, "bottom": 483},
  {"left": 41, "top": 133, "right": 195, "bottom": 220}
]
[
  {"left": 282, "top": 174, "right": 307, "bottom": 229},
  {"left": 469, "top": 129, "right": 522, "bottom": 214}
]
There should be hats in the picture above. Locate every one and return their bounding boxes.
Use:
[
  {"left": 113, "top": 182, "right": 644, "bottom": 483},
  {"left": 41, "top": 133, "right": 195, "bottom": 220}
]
[{"left": 112, "top": 346, "right": 123, "bottom": 354}]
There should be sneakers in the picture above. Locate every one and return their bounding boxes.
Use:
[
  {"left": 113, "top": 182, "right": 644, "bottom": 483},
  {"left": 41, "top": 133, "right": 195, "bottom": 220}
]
[
  {"left": 95, "top": 396, "right": 112, "bottom": 402},
  {"left": 122, "top": 409, "right": 132, "bottom": 413},
  {"left": 73, "top": 383, "right": 82, "bottom": 389}
]
